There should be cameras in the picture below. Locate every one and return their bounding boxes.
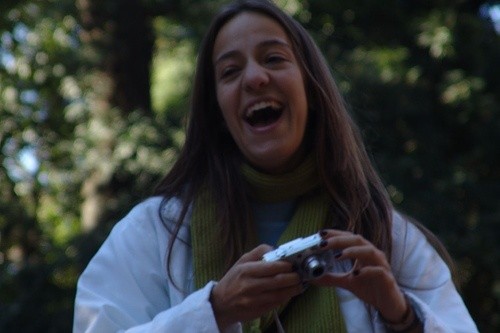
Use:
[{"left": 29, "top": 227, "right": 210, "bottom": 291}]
[{"left": 260, "top": 232, "right": 333, "bottom": 282}]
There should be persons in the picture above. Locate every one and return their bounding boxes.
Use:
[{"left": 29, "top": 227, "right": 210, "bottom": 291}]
[{"left": 71, "top": 0, "right": 478, "bottom": 332}]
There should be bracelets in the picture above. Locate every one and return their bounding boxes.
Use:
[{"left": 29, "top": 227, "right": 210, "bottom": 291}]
[{"left": 379, "top": 290, "right": 409, "bottom": 325}]
